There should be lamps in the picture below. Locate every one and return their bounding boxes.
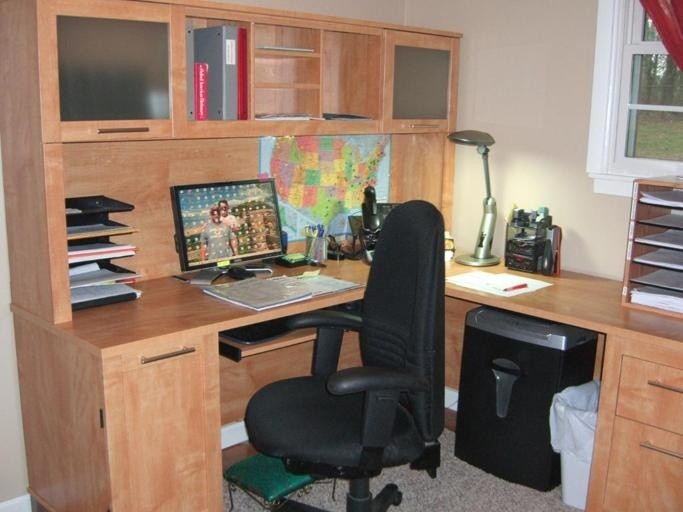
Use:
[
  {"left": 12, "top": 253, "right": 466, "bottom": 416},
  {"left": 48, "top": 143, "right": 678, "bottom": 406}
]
[{"left": 450, "top": 127, "right": 496, "bottom": 269}]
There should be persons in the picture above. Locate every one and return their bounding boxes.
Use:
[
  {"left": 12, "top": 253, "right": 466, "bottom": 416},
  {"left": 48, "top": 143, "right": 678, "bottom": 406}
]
[
  {"left": 217, "top": 199, "right": 241, "bottom": 231},
  {"left": 199, "top": 207, "right": 238, "bottom": 261}
]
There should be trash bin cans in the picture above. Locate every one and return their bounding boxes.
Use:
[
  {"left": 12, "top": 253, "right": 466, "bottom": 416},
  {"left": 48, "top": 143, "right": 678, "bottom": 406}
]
[{"left": 553, "top": 378, "right": 601, "bottom": 510}]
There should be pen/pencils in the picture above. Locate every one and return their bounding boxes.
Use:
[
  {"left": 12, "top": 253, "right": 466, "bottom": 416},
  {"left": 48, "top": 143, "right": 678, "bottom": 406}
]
[
  {"left": 503, "top": 284, "right": 528, "bottom": 291},
  {"left": 172, "top": 275, "right": 190, "bottom": 284},
  {"left": 305, "top": 224, "right": 324, "bottom": 259}
]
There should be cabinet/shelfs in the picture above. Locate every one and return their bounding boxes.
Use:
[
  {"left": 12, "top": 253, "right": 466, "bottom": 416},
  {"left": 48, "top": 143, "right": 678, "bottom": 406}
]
[
  {"left": 620, "top": 175, "right": 682, "bottom": 320},
  {"left": 0, "top": 2, "right": 463, "bottom": 324}
]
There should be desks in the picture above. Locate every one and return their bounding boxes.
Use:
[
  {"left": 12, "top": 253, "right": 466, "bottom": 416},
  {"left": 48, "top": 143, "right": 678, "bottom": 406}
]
[
  {"left": 15, "top": 232, "right": 370, "bottom": 512},
  {"left": 446, "top": 257, "right": 682, "bottom": 512}
]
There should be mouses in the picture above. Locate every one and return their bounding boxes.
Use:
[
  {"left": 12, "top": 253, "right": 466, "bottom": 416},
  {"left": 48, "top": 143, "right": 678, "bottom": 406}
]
[{"left": 342, "top": 303, "right": 358, "bottom": 311}]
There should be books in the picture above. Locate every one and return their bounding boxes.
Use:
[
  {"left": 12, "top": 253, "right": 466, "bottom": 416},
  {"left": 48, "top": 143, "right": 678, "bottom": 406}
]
[
  {"left": 627, "top": 188, "right": 683, "bottom": 314},
  {"left": 256, "top": 44, "right": 312, "bottom": 54},
  {"left": 201, "top": 276, "right": 313, "bottom": 313},
  {"left": 185, "top": 18, "right": 250, "bottom": 121},
  {"left": 254, "top": 112, "right": 312, "bottom": 119},
  {"left": 64, "top": 222, "right": 142, "bottom": 312},
  {"left": 322, "top": 112, "right": 369, "bottom": 119}
]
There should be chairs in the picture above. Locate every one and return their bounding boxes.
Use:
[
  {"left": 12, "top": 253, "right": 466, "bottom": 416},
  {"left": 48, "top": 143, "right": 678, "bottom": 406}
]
[{"left": 243, "top": 200, "right": 446, "bottom": 510}]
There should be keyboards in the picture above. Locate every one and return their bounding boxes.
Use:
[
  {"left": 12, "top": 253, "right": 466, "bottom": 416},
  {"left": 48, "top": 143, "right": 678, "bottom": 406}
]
[{"left": 219, "top": 318, "right": 294, "bottom": 344}]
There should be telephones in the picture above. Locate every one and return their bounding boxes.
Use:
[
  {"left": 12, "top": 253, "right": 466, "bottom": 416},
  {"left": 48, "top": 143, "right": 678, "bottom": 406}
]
[{"left": 360, "top": 187, "right": 403, "bottom": 234}]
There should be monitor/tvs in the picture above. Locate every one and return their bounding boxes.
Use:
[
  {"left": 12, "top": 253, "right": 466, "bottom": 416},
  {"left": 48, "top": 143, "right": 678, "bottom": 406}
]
[{"left": 170, "top": 177, "right": 287, "bottom": 286}]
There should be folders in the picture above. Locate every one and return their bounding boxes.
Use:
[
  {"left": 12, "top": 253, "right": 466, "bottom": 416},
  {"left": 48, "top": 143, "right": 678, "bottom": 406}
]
[{"left": 185, "top": 26, "right": 238, "bottom": 120}]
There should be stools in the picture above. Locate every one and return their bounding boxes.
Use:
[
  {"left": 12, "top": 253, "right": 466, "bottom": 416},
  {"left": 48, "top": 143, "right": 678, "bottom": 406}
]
[{"left": 223, "top": 453, "right": 338, "bottom": 512}]
